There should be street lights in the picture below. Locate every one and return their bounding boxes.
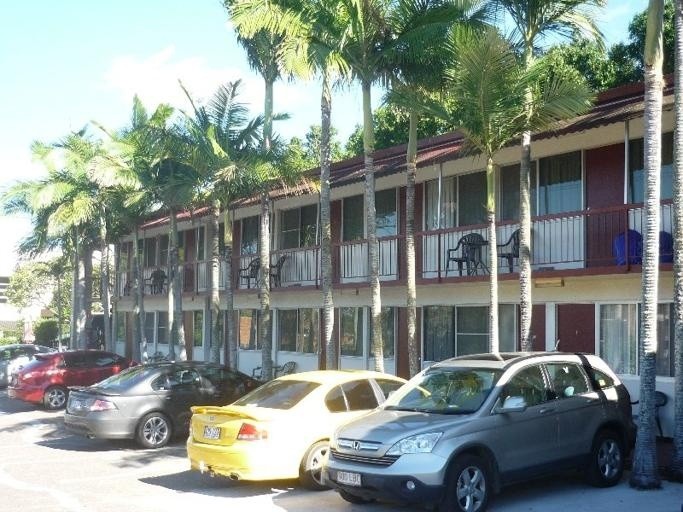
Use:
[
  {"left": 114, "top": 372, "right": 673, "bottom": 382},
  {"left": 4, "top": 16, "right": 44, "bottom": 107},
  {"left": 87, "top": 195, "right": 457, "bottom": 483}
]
[{"left": 31, "top": 268, "right": 62, "bottom": 352}]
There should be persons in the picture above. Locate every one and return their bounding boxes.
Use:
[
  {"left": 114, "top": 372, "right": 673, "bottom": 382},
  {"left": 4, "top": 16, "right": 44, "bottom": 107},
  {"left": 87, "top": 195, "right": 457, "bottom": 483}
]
[{"left": 150, "top": 268, "right": 166, "bottom": 295}]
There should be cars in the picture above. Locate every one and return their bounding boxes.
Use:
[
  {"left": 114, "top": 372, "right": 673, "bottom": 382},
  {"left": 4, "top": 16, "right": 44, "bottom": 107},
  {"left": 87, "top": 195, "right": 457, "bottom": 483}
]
[
  {"left": 62, "top": 360, "right": 261, "bottom": 449},
  {"left": 186, "top": 370, "right": 433, "bottom": 491},
  {"left": 7, "top": 349, "right": 140, "bottom": 410},
  {"left": 0, "top": 344, "right": 57, "bottom": 388}
]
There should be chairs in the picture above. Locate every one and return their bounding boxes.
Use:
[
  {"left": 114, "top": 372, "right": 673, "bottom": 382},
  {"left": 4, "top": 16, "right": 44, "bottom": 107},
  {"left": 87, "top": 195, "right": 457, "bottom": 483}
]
[
  {"left": 142, "top": 269, "right": 167, "bottom": 294},
  {"left": 251, "top": 360, "right": 273, "bottom": 380},
  {"left": 271, "top": 361, "right": 296, "bottom": 377},
  {"left": 630, "top": 389, "right": 670, "bottom": 440},
  {"left": 496, "top": 227, "right": 532, "bottom": 273},
  {"left": 444, "top": 232, "right": 490, "bottom": 278},
  {"left": 237, "top": 254, "right": 261, "bottom": 289},
  {"left": 264, "top": 255, "right": 288, "bottom": 287}
]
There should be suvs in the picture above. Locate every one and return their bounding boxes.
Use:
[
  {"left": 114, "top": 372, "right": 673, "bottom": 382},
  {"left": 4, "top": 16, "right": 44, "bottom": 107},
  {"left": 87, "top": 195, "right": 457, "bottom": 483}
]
[{"left": 321, "top": 351, "right": 634, "bottom": 512}]
